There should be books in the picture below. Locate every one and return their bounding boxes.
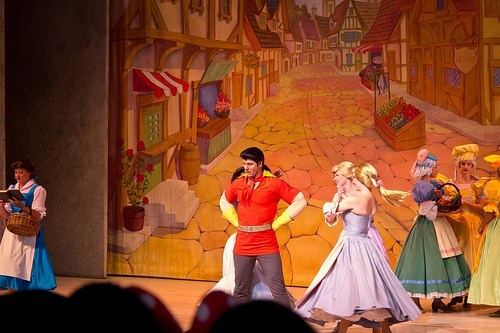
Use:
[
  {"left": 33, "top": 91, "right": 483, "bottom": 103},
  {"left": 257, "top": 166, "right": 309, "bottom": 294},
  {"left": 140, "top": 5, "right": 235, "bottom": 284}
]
[{"left": 0, "top": 188, "right": 26, "bottom": 203}]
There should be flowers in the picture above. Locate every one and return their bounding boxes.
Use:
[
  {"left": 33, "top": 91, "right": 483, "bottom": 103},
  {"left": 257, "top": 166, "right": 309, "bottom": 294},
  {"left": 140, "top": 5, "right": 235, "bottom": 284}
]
[{"left": 434, "top": 189, "right": 460, "bottom": 206}]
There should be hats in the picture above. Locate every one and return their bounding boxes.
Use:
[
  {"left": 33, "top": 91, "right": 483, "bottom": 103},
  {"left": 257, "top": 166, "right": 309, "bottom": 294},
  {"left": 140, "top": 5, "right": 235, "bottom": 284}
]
[{"left": 452, "top": 143, "right": 481, "bottom": 162}]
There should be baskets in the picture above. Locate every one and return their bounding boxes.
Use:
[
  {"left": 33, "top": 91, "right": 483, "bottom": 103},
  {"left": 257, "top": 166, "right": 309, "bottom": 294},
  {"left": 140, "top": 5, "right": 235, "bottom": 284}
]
[
  {"left": 437, "top": 183, "right": 462, "bottom": 213},
  {"left": 6, "top": 205, "right": 41, "bottom": 237}
]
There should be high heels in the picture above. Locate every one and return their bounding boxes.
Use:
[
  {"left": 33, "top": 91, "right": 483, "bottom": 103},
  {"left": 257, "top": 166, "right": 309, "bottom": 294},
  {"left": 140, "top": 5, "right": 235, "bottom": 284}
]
[
  {"left": 431, "top": 298, "right": 455, "bottom": 313},
  {"left": 411, "top": 297, "right": 427, "bottom": 312}
]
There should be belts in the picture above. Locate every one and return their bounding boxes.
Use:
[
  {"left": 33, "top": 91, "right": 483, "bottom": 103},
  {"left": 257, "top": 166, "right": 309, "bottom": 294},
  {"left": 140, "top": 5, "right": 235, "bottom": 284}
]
[{"left": 239, "top": 223, "right": 272, "bottom": 233}]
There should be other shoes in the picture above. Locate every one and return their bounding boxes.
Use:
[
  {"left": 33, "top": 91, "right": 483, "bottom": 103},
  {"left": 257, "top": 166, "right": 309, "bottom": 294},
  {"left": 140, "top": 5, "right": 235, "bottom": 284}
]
[
  {"left": 462, "top": 296, "right": 471, "bottom": 311},
  {"left": 446, "top": 296, "right": 464, "bottom": 306},
  {"left": 487, "top": 309, "right": 500, "bottom": 317}
]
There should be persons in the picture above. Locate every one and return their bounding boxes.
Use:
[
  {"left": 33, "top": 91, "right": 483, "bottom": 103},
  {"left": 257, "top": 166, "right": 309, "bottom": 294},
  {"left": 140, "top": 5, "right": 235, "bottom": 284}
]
[
  {"left": 184, "top": 290, "right": 317, "bottom": 333},
  {"left": 394, "top": 149, "right": 471, "bottom": 314},
  {"left": 219, "top": 147, "right": 307, "bottom": 309},
  {"left": 431, "top": 144, "right": 500, "bottom": 318},
  {"left": 0, "top": 282, "right": 184, "bottom": 333},
  {"left": 206, "top": 165, "right": 298, "bottom": 311},
  {"left": 0, "top": 159, "right": 57, "bottom": 290},
  {"left": 293, "top": 161, "right": 422, "bottom": 333}
]
